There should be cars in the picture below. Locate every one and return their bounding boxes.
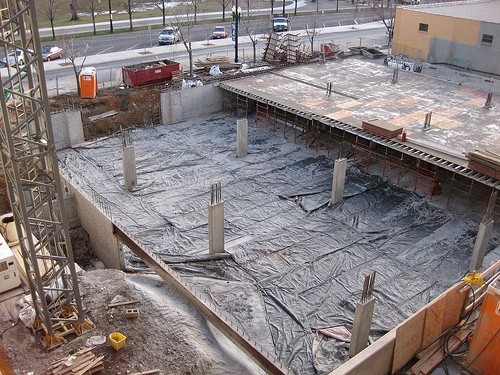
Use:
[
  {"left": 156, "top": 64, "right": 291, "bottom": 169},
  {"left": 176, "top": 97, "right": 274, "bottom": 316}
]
[
  {"left": 33, "top": 45, "right": 64, "bottom": 62},
  {"left": 3, "top": 47, "right": 35, "bottom": 67},
  {"left": 212, "top": 26, "right": 227, "bottom": 39}
]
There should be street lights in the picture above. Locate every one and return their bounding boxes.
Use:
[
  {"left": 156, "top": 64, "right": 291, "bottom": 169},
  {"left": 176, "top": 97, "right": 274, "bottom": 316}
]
[{"left": 231, "top": 5, "right": 242, "bottom": 62}]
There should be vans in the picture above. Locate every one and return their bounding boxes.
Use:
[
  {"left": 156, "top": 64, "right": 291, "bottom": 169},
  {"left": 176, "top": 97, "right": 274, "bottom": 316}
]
[
  {"left": 271, "top": 17, "right": 290, "bottom": 32},
  {"left": 158, "top": 26, "right": 181, "bottom": 44}
]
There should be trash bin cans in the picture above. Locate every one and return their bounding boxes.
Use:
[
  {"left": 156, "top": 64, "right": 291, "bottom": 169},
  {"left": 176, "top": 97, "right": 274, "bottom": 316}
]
[{"left": 122, "top": 59, "right": 181, "bottom": 87}]
